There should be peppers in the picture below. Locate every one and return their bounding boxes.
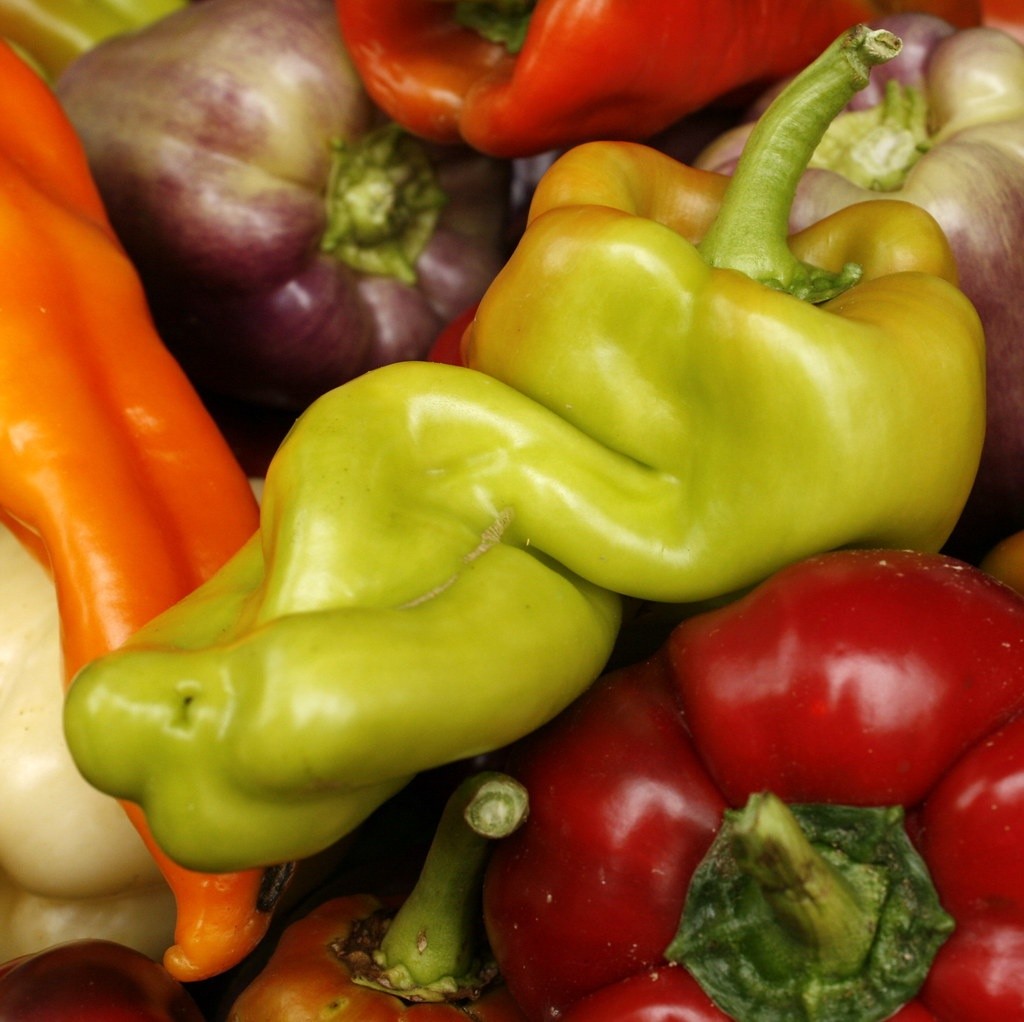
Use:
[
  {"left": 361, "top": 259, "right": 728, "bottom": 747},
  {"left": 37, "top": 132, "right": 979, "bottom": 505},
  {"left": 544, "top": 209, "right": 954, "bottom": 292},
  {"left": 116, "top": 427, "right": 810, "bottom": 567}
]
[{"left": 0, "top": 0, "right": 1024, "bottom": 1022}]
